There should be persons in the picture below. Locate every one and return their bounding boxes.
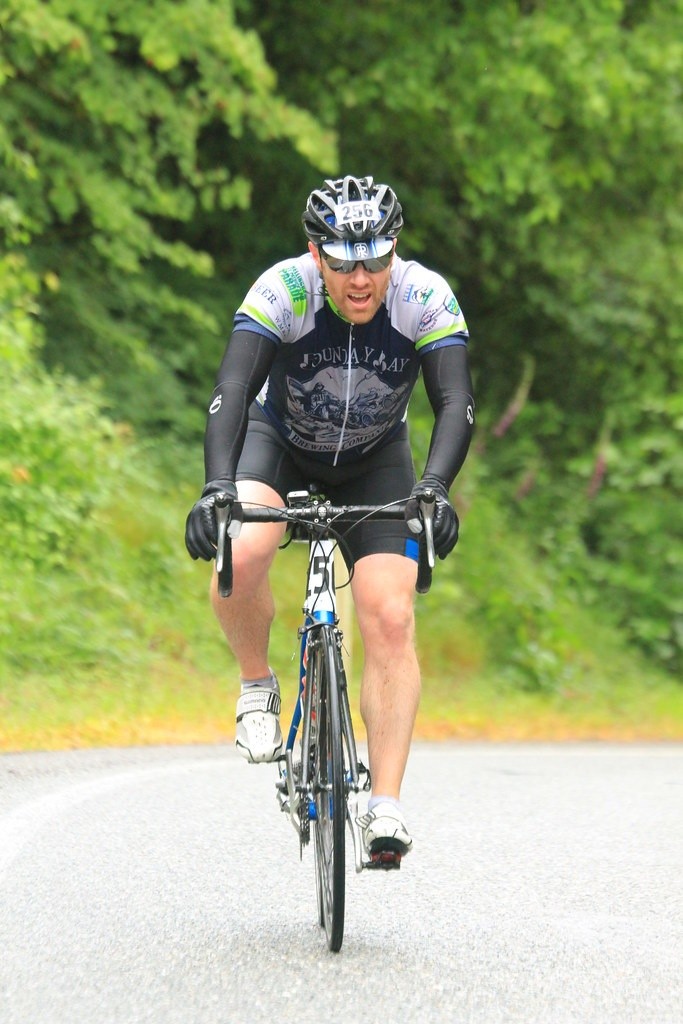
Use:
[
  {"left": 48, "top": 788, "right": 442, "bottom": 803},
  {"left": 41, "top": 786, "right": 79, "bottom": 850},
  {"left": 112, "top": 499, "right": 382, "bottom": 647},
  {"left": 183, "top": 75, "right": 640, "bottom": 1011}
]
[{"left": 183, "top": 176, "right": 477, "bottom": 856}]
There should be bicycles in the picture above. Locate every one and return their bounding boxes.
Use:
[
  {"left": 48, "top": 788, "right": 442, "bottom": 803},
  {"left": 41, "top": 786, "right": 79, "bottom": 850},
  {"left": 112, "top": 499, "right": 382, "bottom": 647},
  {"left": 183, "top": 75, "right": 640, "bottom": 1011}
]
[{"left": 206, "top": 486, "right": 439, "bottom": 955}]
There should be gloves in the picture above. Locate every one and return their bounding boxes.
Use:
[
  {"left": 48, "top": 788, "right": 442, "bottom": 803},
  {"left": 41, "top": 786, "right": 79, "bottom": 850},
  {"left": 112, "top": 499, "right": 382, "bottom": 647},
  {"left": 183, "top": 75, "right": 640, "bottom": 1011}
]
[
  {"left": 185, "top": 491, "right": 244, "bottom": 560},
  {"left": 406, "top": 479, "right": 461, "bottom": 561}
]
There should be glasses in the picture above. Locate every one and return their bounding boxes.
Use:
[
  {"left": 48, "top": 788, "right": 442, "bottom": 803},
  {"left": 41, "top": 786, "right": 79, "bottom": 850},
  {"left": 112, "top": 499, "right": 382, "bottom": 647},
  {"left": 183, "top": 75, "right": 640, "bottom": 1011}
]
[{"left": 319, "top": 244, "right": 396, "bottom": 274}]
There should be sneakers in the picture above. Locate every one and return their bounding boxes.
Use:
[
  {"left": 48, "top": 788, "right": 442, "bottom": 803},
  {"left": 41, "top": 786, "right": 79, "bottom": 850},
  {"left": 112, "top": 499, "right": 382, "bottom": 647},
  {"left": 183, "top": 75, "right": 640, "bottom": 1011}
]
[
  {"left": 236, "top": 666, "right": 283, "bottom": 761},
  {"left": 356, "top": 802, "right": 413, "bottom": 854}
]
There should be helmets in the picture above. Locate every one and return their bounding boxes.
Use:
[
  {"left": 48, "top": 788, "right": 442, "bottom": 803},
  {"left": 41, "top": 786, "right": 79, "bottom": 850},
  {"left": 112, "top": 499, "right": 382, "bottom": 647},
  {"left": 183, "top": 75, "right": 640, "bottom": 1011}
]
[{"left": 302, "top": 176, "right": 403, "bottom": 261}]
